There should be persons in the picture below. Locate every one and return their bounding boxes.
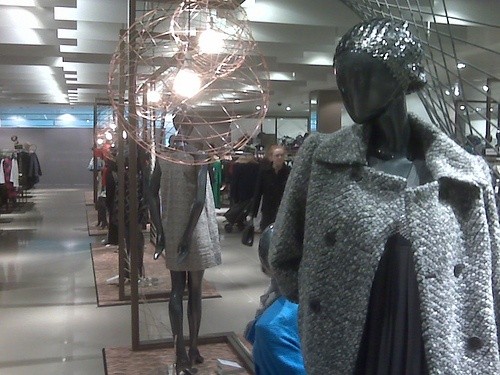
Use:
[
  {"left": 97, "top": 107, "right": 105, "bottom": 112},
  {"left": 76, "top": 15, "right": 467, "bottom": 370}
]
[
  {"left": 242, "top": 145, "right": 293, "bottom": 230},
  {"left": 271, "top": 19, "right": 500, "bottom": 375},
  {"left": 244, "top": 222, "right": 307, "bottom": 375},
  {"left": 96, "top": 154, "right": 110, "bottom": 230},
  {"left": 144, "top": 106, "right": 222, "bottom": 375}
]
